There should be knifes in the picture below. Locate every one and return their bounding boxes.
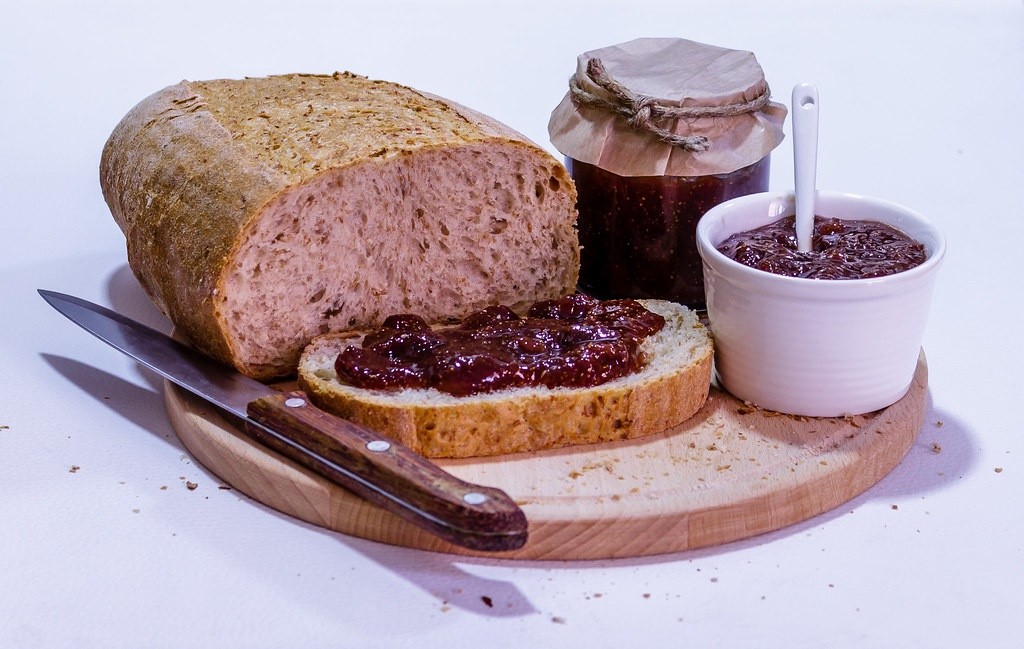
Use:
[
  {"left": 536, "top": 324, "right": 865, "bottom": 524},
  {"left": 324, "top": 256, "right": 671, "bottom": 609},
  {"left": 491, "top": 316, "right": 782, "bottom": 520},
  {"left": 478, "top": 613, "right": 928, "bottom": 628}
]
[{"left": 39, "top": 289, "right": 528, "bottom": 551}]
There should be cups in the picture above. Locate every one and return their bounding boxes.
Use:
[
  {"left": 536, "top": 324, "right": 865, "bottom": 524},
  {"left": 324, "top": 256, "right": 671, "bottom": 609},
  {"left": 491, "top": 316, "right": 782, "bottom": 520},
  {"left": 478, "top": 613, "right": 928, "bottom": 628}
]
[{"left": 697, "top": 191, "right": 945, "bottom": 416}]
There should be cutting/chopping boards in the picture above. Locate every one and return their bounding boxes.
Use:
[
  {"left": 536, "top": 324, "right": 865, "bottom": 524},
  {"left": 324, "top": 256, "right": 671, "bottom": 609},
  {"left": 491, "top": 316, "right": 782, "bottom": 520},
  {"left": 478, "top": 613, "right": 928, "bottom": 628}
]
[{"left": 164, "top": 317, "right": 926, "bottom": 561}]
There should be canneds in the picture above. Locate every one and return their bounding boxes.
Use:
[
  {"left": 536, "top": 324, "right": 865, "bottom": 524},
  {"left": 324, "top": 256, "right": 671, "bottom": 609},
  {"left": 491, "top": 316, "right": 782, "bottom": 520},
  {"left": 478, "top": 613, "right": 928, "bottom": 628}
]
[{"left": 557, "top": 37, "right": 770, "bottom": 319}]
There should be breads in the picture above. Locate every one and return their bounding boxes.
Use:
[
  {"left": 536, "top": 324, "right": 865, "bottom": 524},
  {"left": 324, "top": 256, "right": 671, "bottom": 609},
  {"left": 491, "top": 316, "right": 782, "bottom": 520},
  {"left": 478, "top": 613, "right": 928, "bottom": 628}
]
[
  {"left": 98, "top": 69, "right": 582, "bottom": 383},
  {"left": 297, "top": 300, "right": 716, "bottom": 460}
]
